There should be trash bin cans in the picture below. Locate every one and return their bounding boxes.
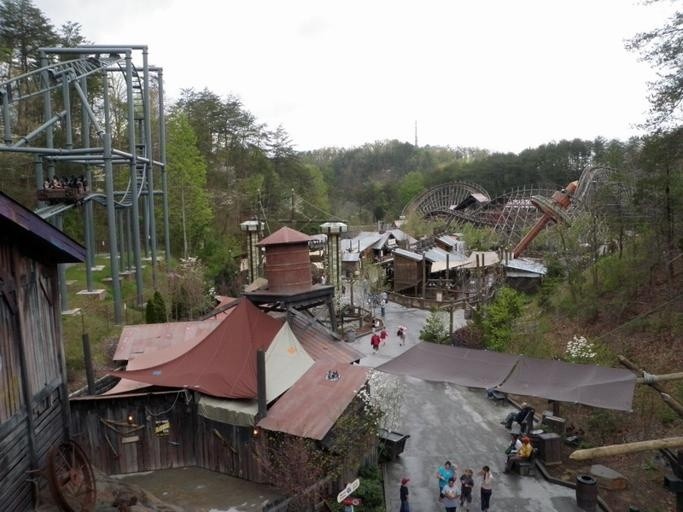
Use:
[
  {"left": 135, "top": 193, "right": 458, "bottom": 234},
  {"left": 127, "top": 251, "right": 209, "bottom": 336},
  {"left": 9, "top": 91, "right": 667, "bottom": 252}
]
[{"left": 576, "top": 474, "right": 597, "bottom": 512}]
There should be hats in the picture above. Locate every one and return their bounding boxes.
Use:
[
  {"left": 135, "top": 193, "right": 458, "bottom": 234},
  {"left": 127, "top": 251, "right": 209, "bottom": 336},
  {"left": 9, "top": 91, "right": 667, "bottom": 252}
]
[
  {"left": 402, "top": 478, "right": 410, "bottom": 485},
  {"left": 521, "top": 437, "right": 529, "bottom": 443}
]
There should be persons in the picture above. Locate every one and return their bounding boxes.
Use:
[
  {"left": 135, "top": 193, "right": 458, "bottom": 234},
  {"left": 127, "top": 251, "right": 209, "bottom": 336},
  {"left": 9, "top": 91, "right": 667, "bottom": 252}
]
[
  {"left": 459, "top": 469, "right": 474, "bottom": 512},
  {"left": 320, "top": 271, "right": 328, "bottom": 285},
  {"left": 504, "top": 435, "right": 522, "bottom": 464},
  {"left": 502, "top": 436, "right": 533, "bottom": 474},
  {"left": 398, "top": 323, "right": 407, "bottom": 347},
  {"left": 436, "top": 460, "right": 458, "bottom": 503},
  {"left": 442, "top": 477, "right": 461, "bottom": 512},
  {"left": 380, "top": 299, "right": 386, "bottom": 317},
  {"left": 43, "top": 175, "right": 88, "bottom": 209},
  {"left": 370, "top": 317, "right": 389, "bottom": 353},
  {"left": 476, "top": 465, "right": 494, "bottom": 512},
  {"left": 400, "top": 478, "right": 410, "bottom": 512}
]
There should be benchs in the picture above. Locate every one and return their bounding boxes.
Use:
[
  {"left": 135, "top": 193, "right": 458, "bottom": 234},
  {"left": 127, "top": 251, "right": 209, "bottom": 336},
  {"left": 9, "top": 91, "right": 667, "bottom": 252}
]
[
  {"left": 518, "top": 448, "right": 538, "bottom": 476},
  {"left": 493, "top": 393, "right": 505, "bottom": 406},
  {"left": 510, "top": 410, "right": 535, "bottom": 440}
]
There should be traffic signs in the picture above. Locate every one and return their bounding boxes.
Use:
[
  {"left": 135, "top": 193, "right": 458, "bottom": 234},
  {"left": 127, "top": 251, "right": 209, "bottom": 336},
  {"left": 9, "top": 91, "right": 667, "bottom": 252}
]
[
  {"left": 342, "top": 497, "right": 361, "bottom": 508},
  {"left": 336, "top": 477, "right": 361, "bottom": 505}
]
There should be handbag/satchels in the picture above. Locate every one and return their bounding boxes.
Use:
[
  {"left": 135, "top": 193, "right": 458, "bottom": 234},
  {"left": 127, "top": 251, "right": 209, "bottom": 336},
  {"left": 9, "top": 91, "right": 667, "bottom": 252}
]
[{"left": 505, "top": 444, "right": 516, "bottom": 454}]
